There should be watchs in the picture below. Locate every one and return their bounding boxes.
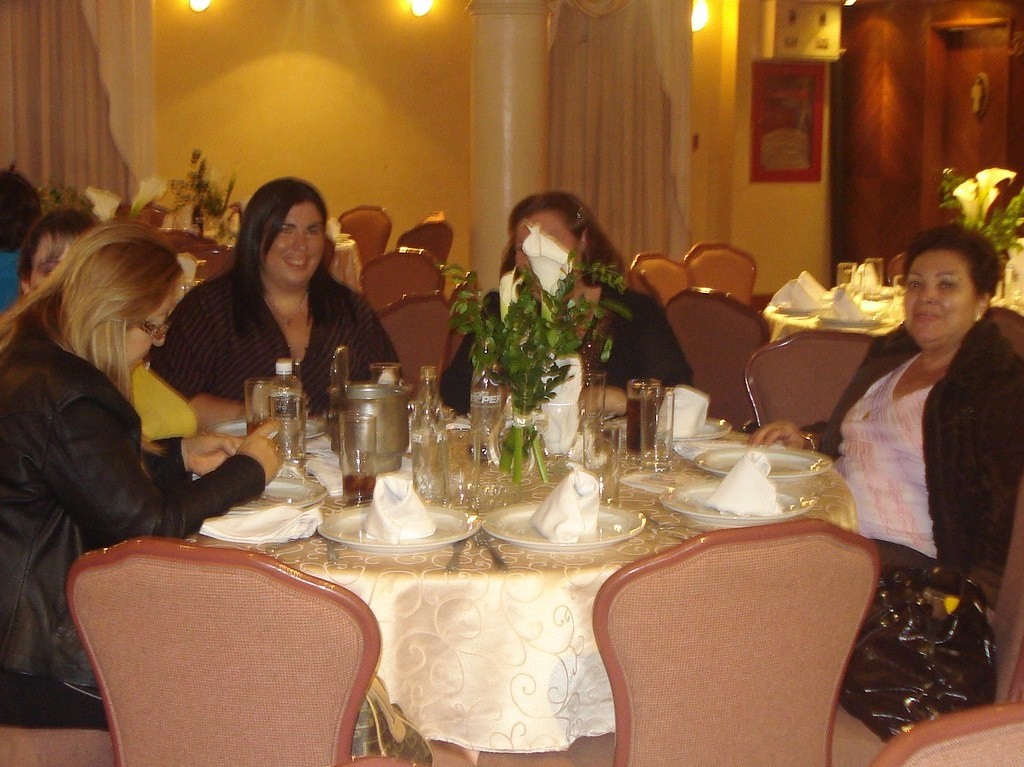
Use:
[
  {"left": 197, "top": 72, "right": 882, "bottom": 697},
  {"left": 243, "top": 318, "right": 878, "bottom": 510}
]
[{"left": 803, "top": 430, "right": 818, "bottom": 452}]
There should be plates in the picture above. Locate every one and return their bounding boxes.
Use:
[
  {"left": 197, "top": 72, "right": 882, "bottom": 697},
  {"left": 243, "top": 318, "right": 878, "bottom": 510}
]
[
  {"left": 191, "top": 476, "right": 329, "bottom": 515},
  {"left": 660, "top": 485, "right": 817, "bottom": 527},
  {"left": 317, "top": 504, "right": 481, "bottom": 555},
  {"left": 775, "top": 303, "right": 824, "bottom": 316},
  {"left": 693, "top": 447, "right": 833, "bottom": 485},
  {"left": 482, "top": 500, "right": 646, "bottom": 555},
  {"left": 656, "top": 413, "right": 732, "bottom": 442},
  {"left": 815, "top": 314, "right": 882, "bottom": 327},
  {"left": 205, "top": 416, "right": 327, "bottom": 440}
]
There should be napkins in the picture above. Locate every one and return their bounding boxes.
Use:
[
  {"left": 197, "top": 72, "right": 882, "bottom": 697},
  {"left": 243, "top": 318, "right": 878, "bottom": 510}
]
[
  {"left": 526, "top": 471, "right": 603, "bottom": 549},
  {"left": 849, "top": 259, "right": 875, "bottom": 295},
  {"left": 825, "top": 286, "right": 860, "bottom": 325},
  {"left": 772, "top": 280, "right": 819, "bottom": 310},
  {"left": 703, "top": 448, "right": 785, "bottom": 523},
  {"left": 322, "top": 215, "right": 342, "bottom": 240},
  {"left": 199, "top": 500, "right": 323, "bottom": 549},
  {"left": 651, "top": 383, "right": 713, "bottom": 441},
  {"left": 794, "top": 269, "right": 829, "bottom": 301},
  {"left": 302, "top": 451, "right": 417, "bottom": 501},
  {"left": 362, "top": 474, "right": 437, "bottom": 544}
]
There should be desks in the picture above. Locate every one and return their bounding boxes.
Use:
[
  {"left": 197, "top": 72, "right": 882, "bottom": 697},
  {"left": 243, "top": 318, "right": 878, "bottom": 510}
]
[
  {"left": 180, "top": 406, "right": 860, "bottom": 766},
  {"left": 317, "top": 214, "right": 370, "bottom": 301}
]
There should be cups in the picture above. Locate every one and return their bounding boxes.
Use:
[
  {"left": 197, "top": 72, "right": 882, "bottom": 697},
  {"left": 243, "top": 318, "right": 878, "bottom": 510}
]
[
  {"left": 339, "top": 412, "right": 377, "bottom": 508},
  {"left": 327, "top": 383, "right": 411, "bottom": 474},
  {"left": 577, "top": 370, "right": 607, "bottom": 433},
  {"left": 438, "top": 414, "right": 481, "bottom": 513},
  {"left": 582, "top": 421, "right": 621, "bottom": 509},
  {"left": 626, "top": 378, "right": 675, "bottom": 474},
  {"left": 244, "top": 376, "right": 273, "bottom": 437},
  {"left": 369, "top": 362, "right": 402, "bottom": 386},
  {"left": 837, "top": 262, "right": 858, "bottom": 294},
  {"left": 864, "top": 257, "right": 884, "bottom": 292}
]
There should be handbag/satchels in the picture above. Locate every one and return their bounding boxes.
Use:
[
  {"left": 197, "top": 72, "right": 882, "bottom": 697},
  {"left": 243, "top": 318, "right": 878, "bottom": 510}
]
[{"left": 840, "top": 560, "right": 996, "bottom": 739}]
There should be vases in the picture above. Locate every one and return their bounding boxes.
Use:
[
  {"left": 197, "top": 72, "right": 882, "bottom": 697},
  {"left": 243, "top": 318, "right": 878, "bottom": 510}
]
[{"left": 469, "top": 393, "right": 569, "bottom": 515}]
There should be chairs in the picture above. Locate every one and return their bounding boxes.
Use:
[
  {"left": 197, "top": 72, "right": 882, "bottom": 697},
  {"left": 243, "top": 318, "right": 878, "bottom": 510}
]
[{"left": 0, "top": 202, "right": 1024, "bottom": 767}]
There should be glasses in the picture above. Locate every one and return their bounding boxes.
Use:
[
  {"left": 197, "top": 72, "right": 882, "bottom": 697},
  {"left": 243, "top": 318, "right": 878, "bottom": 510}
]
[{"left": 130, "top": 316, "right": 172, "bottom": 341}]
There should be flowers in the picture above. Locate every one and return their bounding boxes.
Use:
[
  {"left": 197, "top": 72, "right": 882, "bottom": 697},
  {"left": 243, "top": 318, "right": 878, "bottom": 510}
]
[
  {"left": 430, "top": 219, "right": 637, "bottom": 490},
  {"left": 935, "top": 160, "right": 1024, "bottom": 267},
  {"left": 33, "top": 176, "right": 168, "bottom": 233},
  {"left": 167, "top": 144, "right": 244, "bottom": 253}
]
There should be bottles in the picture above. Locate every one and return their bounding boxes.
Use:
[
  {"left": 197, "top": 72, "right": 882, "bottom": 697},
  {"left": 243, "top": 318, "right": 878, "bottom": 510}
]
[
  {"left": 268, "top": 358, "right": 302, "bottom": 461},
  {"left": 471, "top": 338, "right": 510, "bottom": 463},
  {"left": 410, "top": 366, "right": 447, "bottom": 507}
]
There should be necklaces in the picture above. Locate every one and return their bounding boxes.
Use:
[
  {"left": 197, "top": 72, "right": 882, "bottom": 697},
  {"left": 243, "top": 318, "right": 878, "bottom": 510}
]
[{"left": 264, "top": 288, "right": 305, "bottom": 328}]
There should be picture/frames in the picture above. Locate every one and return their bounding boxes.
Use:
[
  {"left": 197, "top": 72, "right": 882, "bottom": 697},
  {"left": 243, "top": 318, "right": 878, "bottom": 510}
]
[{"left": 747, "top": 56, "right": 829, "bottom": 188}]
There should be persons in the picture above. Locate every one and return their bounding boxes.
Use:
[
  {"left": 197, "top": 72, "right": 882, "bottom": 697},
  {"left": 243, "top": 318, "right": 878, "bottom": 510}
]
[
  {"left": 439, "top": 193, "right": 696, "bottom": 418},
  {"left": 0, "top": 164, "right": 401, "bottom": 734},
  {"left": 744, "top": 226, "right": 1024, "bottom": 767}
]
